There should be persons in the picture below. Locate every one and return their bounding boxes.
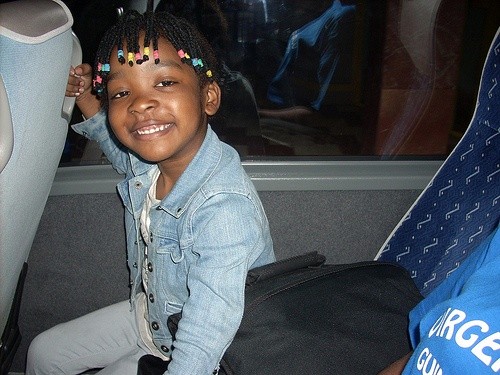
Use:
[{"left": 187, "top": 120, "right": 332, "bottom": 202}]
[
  {"left": 379, "top": 219, "right": 500, "bottom": 374},
  {"left": 148, "top": 1, "right": 383, "bottom": 157},
  {"left": 26, "top": 9, "right": 278, "bottom": 375}
]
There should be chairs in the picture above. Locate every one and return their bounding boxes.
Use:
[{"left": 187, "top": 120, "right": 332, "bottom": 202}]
[
  {"left": 0, "top": 0, "right": 82, "bottom": 343},
  {"left": 372, "top": 24, "right": 498, "bottom": 299}
]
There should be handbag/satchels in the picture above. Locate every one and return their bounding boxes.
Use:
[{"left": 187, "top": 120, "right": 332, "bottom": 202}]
[{"left": 168, "top": 250, "right": 427, "bottom": 375}]
